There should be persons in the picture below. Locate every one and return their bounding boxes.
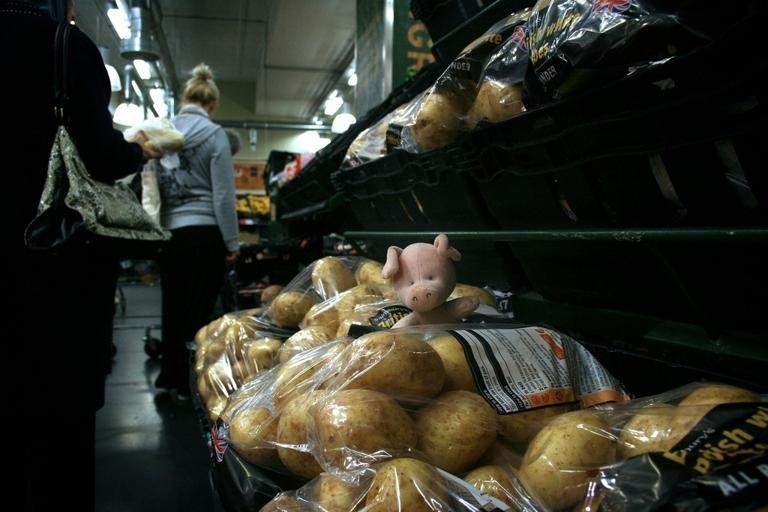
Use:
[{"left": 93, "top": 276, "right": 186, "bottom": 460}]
[
  {"left": 0, "top": 0, "right": 165, "bottom": 512},
  {"left": 151, "top": 61, "right": 242, "bottom": 403},
  {"left": 223, "top": 128, "right": 242, "bottom": 157}
]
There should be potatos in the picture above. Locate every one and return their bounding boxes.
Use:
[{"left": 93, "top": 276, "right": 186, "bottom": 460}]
[
  {"left": 193, "top": 256, "right": 763, "bottom": 511},
  {"left": 337, "top": 80, "right": 520, "bottom": 168}
]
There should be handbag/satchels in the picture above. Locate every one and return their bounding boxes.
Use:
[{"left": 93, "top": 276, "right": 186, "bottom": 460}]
[
  {"left": 119, "top": 155, "right": 161, "bottom": 222},
  {"left": 25, "top": 125, "right": 174, "bottom": 262}
]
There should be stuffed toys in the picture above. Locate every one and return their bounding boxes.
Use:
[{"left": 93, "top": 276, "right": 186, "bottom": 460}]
[{"left": 380, "top": 233, "right": 481, "bottom": 329}]
